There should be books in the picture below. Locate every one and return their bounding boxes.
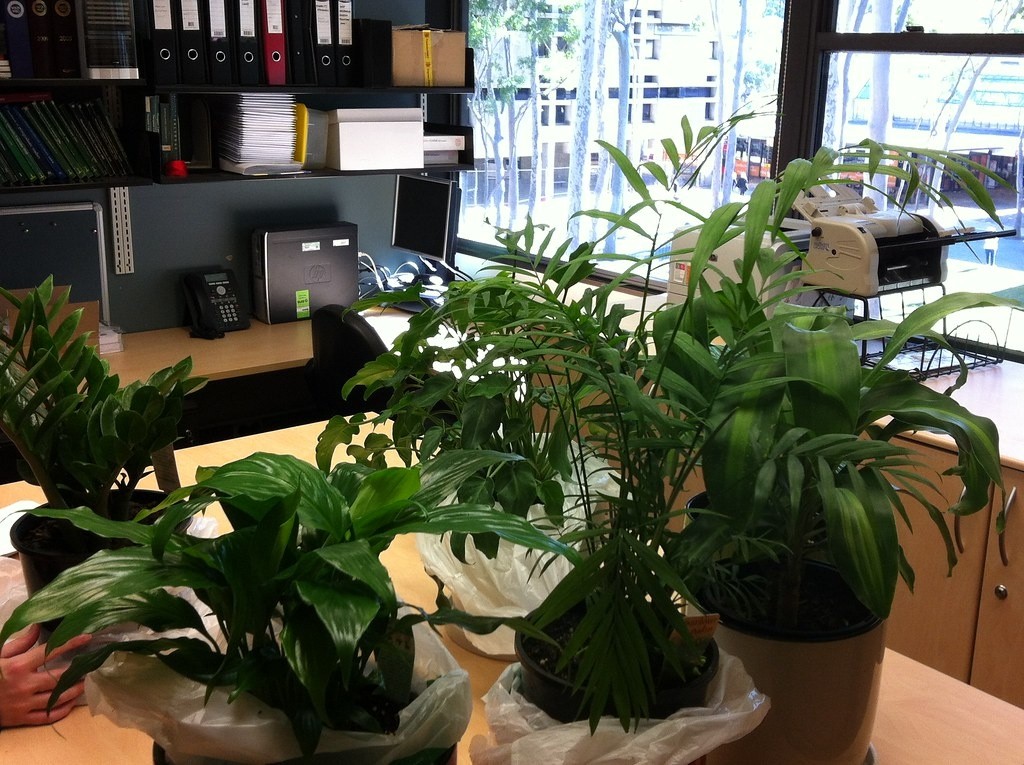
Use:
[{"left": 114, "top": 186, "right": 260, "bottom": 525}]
[
  {"left": 716, "top": 135, "right": 1024, "bottom": 204},
  {"left": 0, "top": 94, "right": 180, "bottom": 188}
]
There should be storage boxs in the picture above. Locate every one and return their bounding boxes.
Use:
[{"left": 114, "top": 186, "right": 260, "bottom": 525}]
[
  {"left": 0, "top": 284, "right": 100, "bottom": 367},
  {"left": 391, "top": 24, "right": 466, "bottom": 87},
  {"left": 423, "top": 150, "right": 458, "bottom": 164},
  {"left": 423, "top": 136, "right": 465, "bottom": 151},
  {"left": 328, "top": 122, "right": 425, "bottom": 170}
]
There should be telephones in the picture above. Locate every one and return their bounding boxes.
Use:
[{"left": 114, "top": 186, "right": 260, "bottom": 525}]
[{"left": 181, "top": 267, "right": 252, "bottom": 340}]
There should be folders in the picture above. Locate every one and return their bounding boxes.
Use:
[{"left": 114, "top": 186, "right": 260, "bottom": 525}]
[{"left": 147, "top": 0, "right": 356, "bottom": 88}]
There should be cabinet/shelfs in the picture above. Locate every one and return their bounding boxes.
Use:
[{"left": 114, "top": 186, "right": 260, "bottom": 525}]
[
  {"left": 526, "top": 293, "right": 1023, "bottom": 709},
  {"left": 0, "top": 0, "right": 477, "bottom": 195}
]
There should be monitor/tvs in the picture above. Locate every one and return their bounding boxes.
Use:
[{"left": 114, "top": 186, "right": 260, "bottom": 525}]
[{"left": 390, "top": 173, "right": 458, "bottom": 261}]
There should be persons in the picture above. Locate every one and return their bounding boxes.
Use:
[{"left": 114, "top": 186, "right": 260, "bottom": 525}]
[{"left": 0, "top": 624, "right": 94, "bottom": 733}]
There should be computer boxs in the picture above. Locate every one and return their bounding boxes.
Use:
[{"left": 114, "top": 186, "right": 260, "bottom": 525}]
[{"left": 253, "top": 219, "right": 359, "bottom": 324}]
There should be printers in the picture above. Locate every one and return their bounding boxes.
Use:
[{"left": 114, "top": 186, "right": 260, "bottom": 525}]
[
  {"left": 791, "top": 175, "right": 974, "bottom": 297},
  {"left": 666, "top": 216, "right": 855, "bottom": 347}
]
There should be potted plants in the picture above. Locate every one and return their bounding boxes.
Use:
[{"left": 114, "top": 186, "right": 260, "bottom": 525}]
[{"left": 0, "top": 105, "right": 1021, "bottom": 765}]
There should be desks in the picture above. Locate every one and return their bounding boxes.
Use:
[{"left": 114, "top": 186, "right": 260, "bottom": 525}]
[
  {"left": 78, "top": 304, "right": 415, "bottom": 397},
  {"left": 1, "top": 412, "right": 1024, "bottom": 763}
]
